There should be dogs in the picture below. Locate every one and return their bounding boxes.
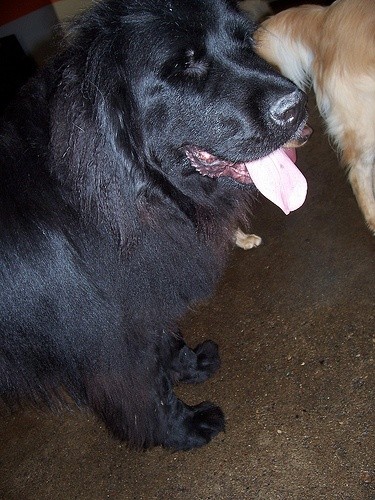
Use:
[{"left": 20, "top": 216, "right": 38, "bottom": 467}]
[
  {"left": 253, "top": 0, "right": 375, "bottom": 234},
  {"left": 0, "top": 0, "right": 314, "bottom": 456}
]
